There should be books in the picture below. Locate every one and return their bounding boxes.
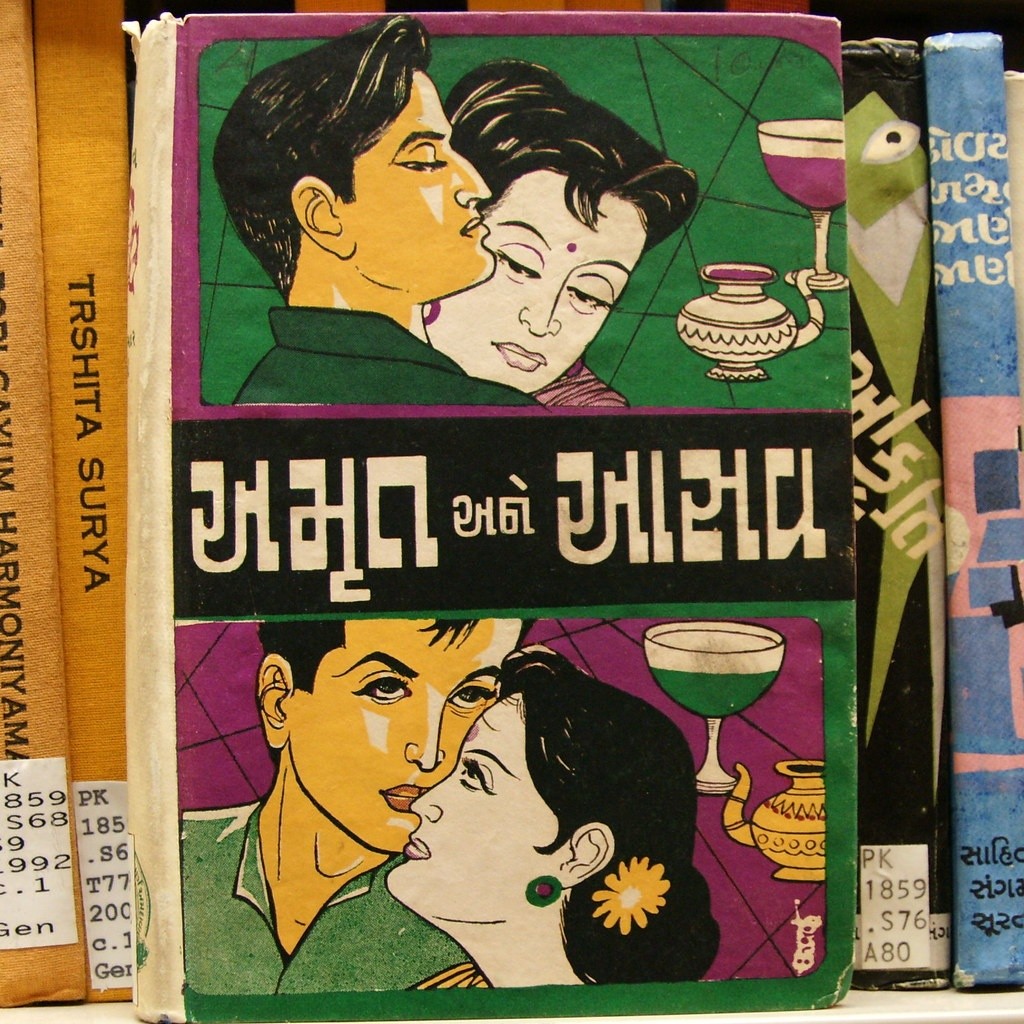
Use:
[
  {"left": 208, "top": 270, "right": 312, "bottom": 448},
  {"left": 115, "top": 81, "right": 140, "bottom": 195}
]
[{"left": 1, "top": 1, "right": 1024, "bottom": 1024}]
